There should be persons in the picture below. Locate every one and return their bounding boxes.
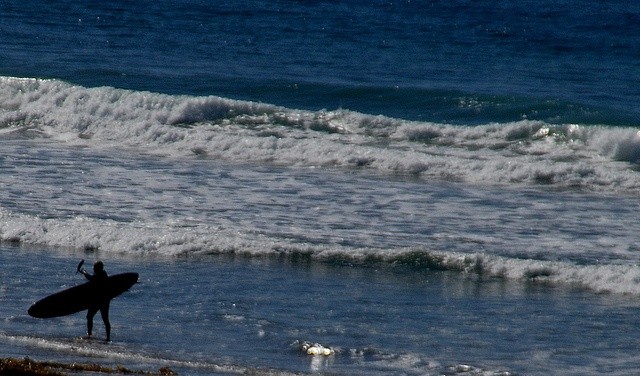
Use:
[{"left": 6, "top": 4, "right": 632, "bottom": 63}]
[{"left": 76, "top": 259, "right": 111, "bottom": 342}]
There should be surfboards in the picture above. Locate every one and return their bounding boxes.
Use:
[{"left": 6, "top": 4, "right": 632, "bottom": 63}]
[{"left": 28, "top": 273, "right": 138, "bottom": 319}]
